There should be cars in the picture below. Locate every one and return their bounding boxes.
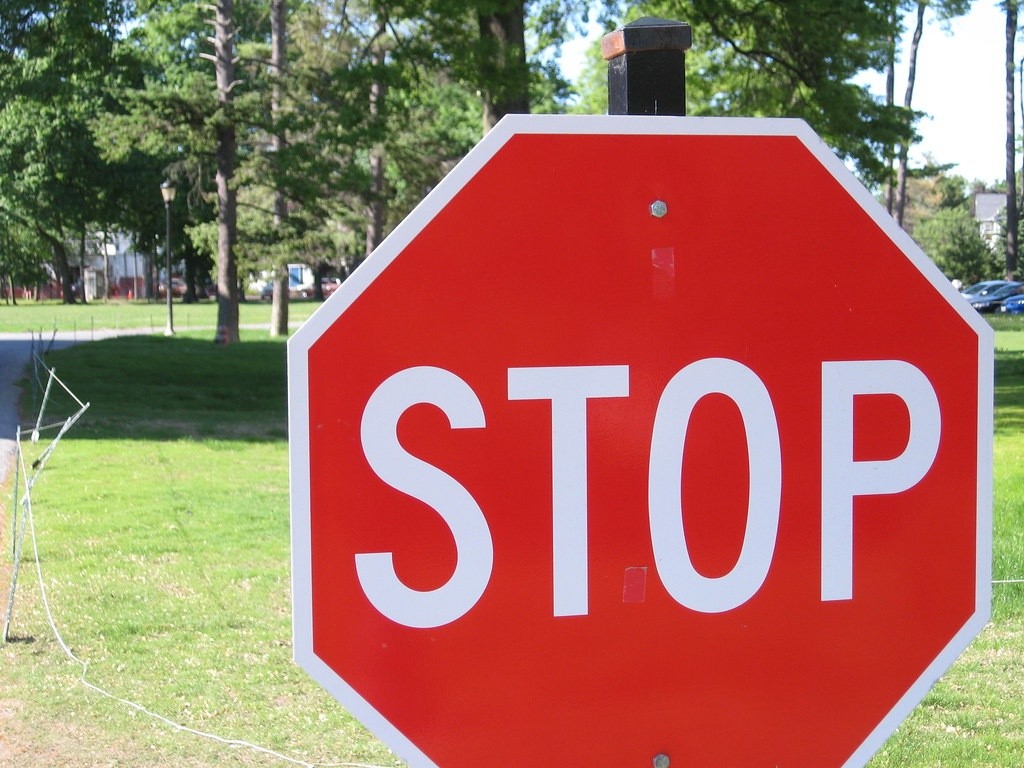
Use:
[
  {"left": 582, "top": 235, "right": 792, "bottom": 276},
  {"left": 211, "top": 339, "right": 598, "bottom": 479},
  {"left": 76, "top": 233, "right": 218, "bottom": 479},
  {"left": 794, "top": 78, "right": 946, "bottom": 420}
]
[
  {"left": 1000, "top": 294, "right": 1024, "bottom": 314},
  {"left": 967, "top": 280, "right": 1023, "bottom": 314},
  {"left": 958, "top": 282, "right": 1018, "bottom": 301},
  {"left": 159, "top": 274, "right": 202, "bottom": 299}
]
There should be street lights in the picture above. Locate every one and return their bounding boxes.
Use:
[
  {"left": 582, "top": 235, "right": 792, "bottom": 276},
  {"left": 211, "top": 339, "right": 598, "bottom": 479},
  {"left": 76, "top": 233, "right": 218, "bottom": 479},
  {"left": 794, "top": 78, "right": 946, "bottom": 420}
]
[{"left": 159, "top": 177, "right": 178, "bottom": 337}]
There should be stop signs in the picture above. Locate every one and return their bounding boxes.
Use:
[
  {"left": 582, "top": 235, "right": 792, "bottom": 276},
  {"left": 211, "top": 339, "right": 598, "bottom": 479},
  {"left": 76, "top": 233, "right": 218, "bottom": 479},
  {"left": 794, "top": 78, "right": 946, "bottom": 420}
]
[{"left": 283, "top": 116, "right": 994, "bottom": 767}]
